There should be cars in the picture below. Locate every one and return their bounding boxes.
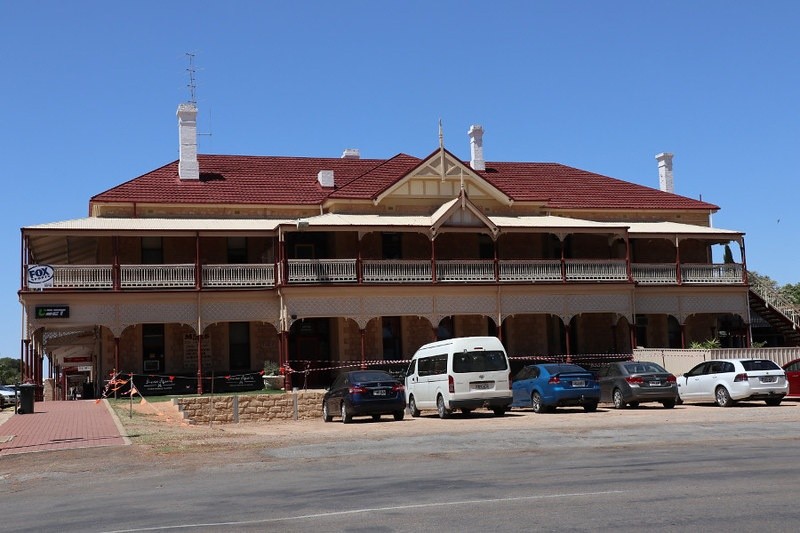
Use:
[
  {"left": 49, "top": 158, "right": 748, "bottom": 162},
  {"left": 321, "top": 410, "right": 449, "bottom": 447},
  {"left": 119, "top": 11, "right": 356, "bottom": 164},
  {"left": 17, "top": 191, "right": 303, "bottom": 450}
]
[
  {"left": 596, "top": 361, "right": 678, "bottom": 410},
  {"left": 781, "top": 358, "right": 800, "bottom": 397},
  {"left": 675, "top": 358, "right": 790, "bottom": 408},
  {"left": 322, "top": 369, "right": 406, "bottom": 424},
  {"left": 511, "top": 363, "right": 601, "bottom": 413},
  {"left": 0, "top": 385, "right": 21, "bottom": 412}
]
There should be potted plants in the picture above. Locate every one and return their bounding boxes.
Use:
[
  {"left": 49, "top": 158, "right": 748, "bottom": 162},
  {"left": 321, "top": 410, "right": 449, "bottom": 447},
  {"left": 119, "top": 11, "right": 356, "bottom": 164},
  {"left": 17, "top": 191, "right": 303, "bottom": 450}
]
[{"left": 261, "top": 361, "right": 284, "bottom": 390}]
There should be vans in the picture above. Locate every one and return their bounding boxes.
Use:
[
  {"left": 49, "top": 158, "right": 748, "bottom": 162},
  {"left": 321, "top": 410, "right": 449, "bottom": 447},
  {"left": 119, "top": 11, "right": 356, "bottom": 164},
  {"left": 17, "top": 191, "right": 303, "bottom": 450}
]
[{"left": 400, "top": 335, "right": 514, "bottom": 420}]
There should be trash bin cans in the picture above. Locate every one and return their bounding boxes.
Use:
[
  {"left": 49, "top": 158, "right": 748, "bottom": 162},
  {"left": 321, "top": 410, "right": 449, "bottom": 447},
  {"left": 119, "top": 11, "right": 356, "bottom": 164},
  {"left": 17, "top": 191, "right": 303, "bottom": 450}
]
[{"left": 18, "top": 384, "right": 36, "bottom": 414}]
